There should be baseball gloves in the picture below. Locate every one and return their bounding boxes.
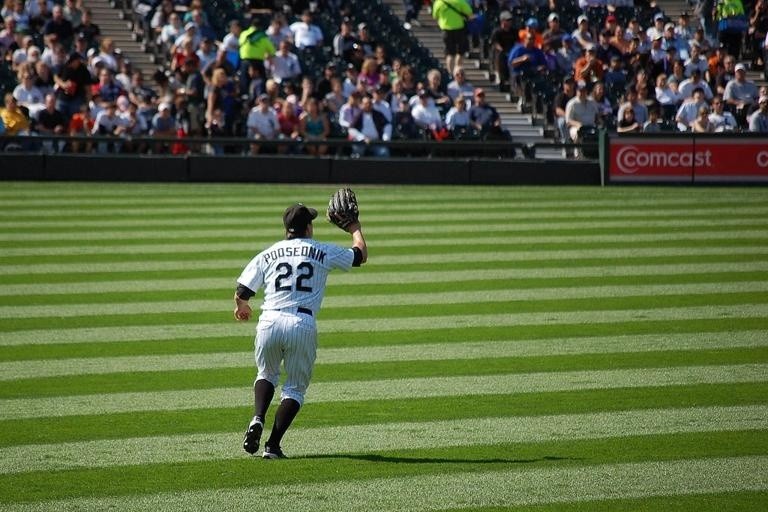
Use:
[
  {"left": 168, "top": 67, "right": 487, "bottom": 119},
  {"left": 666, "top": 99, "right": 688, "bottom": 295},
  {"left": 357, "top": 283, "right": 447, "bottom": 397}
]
[{"left": 326, "top": 189, "right": 361, "bottom": 234}]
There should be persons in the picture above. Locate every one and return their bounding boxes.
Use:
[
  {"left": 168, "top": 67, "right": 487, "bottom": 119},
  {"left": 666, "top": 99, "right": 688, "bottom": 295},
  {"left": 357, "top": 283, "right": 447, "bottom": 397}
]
[
  {"left": 233, "top": 188, "right": 368, "bottom": 460},
  {"left": 0, "top": 2, "right": 768, "bottom": 158}
]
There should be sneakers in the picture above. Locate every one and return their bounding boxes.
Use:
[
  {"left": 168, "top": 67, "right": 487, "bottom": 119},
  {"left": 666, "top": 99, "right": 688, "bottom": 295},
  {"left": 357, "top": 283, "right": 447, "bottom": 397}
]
[
  {"left": 243, "top": 416, "right": 264, "bottom": 454},
  {"left": 262, "top": 442, "right": 287, "bottom": 458}
]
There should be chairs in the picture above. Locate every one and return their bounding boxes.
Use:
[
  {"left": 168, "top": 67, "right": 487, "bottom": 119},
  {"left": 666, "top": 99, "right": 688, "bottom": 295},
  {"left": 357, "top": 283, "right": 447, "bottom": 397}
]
[{"left": 0, "top": 0, "right": 768, "bottom": 159}]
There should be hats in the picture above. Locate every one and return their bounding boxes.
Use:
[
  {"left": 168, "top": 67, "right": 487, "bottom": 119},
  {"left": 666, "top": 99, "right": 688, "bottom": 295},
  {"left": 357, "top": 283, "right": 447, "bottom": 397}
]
[
  {"left": 283, "top": 205, "right": 318, "bottom": 233},
  {"left": 499, "top": 11, "right": 768, "bottom": 125}
]
